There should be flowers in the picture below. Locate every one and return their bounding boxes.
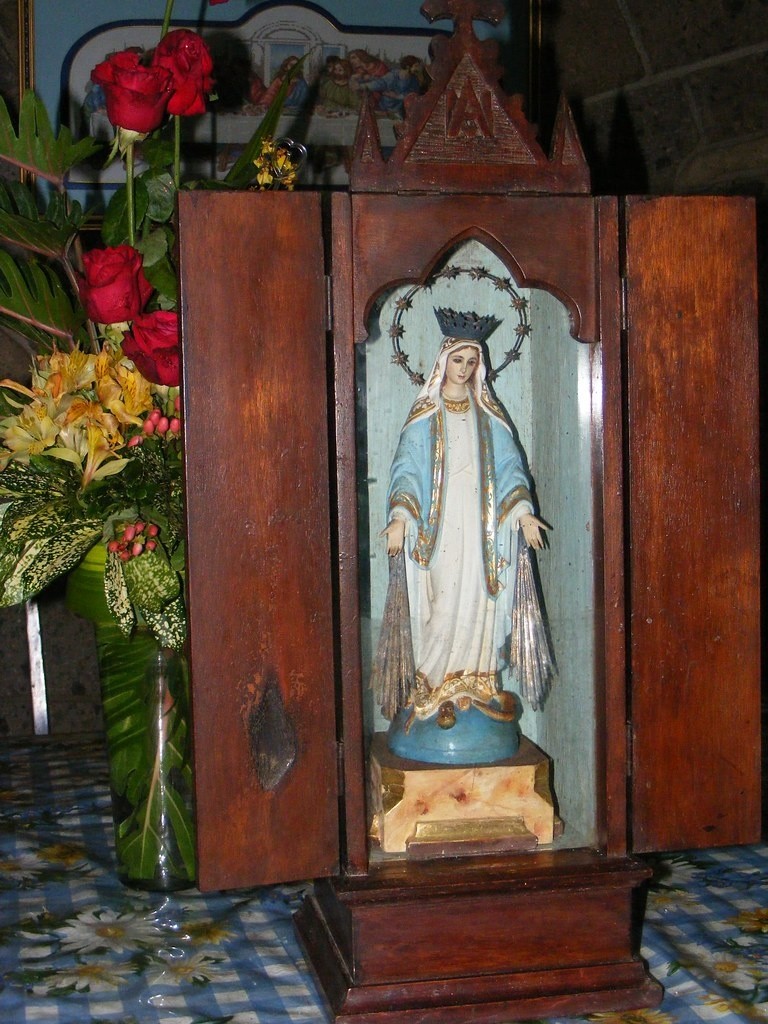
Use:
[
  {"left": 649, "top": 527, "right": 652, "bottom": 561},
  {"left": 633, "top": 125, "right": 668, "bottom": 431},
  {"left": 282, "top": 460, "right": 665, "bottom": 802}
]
[{"left": 0, "top": 0, "right": 316, "bottom": 653}]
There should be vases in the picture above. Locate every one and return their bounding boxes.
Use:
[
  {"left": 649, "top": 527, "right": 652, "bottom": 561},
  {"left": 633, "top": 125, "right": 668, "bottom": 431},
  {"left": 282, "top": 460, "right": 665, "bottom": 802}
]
[{"left": 94, "top": 571, "right": 198, "bottom": 893}]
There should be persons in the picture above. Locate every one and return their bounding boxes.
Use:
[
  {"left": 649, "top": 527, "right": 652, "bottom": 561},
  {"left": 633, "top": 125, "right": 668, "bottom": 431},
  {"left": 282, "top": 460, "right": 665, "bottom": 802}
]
[{"left": 367, "top": 305, "right": 557, "bottom": 730}]
